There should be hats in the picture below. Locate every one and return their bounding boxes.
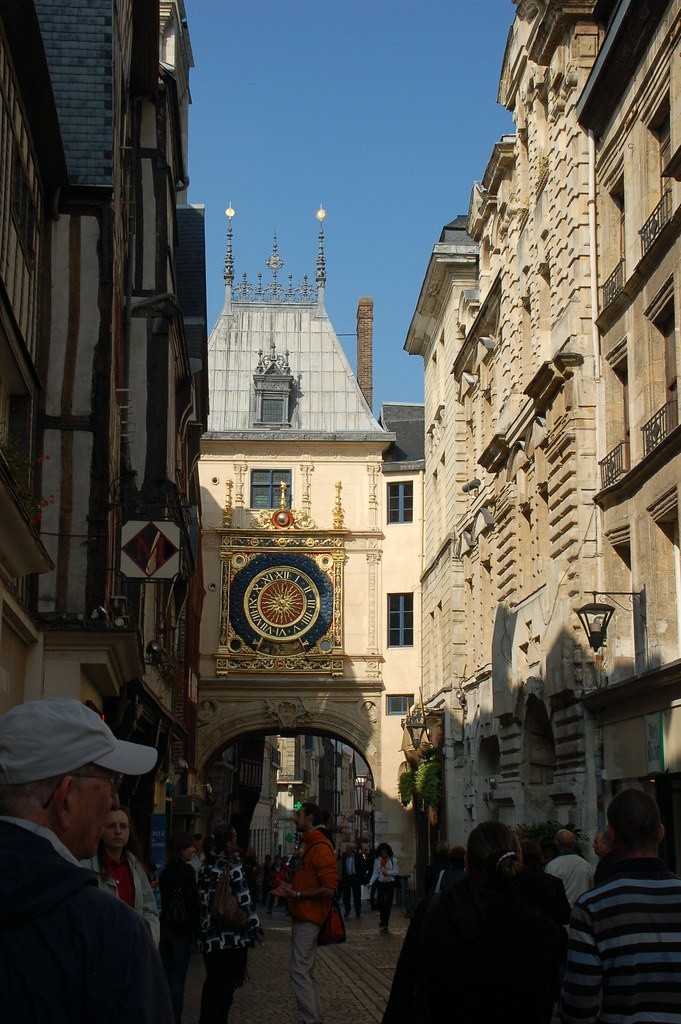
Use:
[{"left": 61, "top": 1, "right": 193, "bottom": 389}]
[{"left": 0, "top": 699, "right": 158, "bottom": 786}]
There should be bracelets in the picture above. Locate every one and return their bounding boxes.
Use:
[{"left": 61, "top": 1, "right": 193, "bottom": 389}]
[
  {"left": 293, "top": 890, "right": 296, "bottom": 899},
  {"left": 301, "top": 891, "right": 304, "bottom": 899},
  {"left": 297, "top": 891, "right": 301, "bottom": 899}
]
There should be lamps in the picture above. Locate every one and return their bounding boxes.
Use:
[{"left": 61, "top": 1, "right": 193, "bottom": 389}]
[
  {"left": 196, "top": 783, "right": 212, "bottom": 793},
  {"left": 494, "top": 712, "right": 513, "bottom": 724},
  {"left": 145, "top": 640, "right": 160, "bottom": 656},
  {"left": 109, "top": 595, "right": 128, "bottom": 618},
  {"left": 287, "top": 785, "right": 292, "bottom": 792},
  {"left": 455, "top": 691, "right": 465, "bottom": 706},
  {"left": 159, "top": 772, "right": 175, "bottom": 784},
  {"left": 111, "top": 615, "right": 131, "bottom": 629},
  {"left": 575, "top": 591, "right": 641, "bottom": 653},
  {"left": 89, "top": 606, "right": 106, "bottom": 623},
  {"left": 145, "top": 652, "right": 167, "bottom": 668},
  {"left": 113, "top": 616, "right": 123, "bottom": 629}
]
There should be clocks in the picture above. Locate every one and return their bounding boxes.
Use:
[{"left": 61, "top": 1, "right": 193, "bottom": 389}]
[{"left": 243, "top": 566, "right": 321, "bottom": 642}]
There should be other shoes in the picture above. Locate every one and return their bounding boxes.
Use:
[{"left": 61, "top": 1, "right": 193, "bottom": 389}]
[
  {"left": 344, "top": 911, "right": 350, "bottom": 917},
  {"left": 266, "top": 911, "right": 272, "bottom": 915},
  {"left": 379, "top": 921, "right": 388, "bottom": 933}
]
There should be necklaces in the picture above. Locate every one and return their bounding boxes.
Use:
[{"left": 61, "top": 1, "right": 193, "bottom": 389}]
[{"left": 111, "top": 867, "right": 120, "bottom": 884}]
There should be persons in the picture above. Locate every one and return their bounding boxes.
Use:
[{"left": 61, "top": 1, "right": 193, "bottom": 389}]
[
  {"left": 76, "top": 804, "right": 161, "bottom": 950},
  {"left": 271, "top": 803, "right": 338, "bottom": 1024},
  {"left": 0, "top": 698, "right": 176, "bottom": 1024},
  {"left": 239, "top": 843, "right": 399, "bottom": 930},
  {"left": 381, "top": 788, "right": 681, "bottom": 1024},
  {"left": 159, "top": 823, "right": 265, "bottom": 1024}
]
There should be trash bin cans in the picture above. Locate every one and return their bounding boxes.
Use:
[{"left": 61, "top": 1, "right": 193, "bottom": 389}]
[{"left": 395, "top": 875, "right": 411, "bottom": 906}]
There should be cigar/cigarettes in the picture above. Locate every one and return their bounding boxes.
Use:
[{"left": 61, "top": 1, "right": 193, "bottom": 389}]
[{"left": 261, "top": 942, "right": 265, "bottom": 946}]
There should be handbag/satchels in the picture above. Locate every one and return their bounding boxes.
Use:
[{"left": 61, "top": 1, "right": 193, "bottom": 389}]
[
  {"left": 317, "top": 900, "right": 346, "bottom": 945},
  {"left": 394, "top": 875, "right": 402, "bottom": 889},
  {"left": 159, "top": 895, "right": 193, "bottom": 929}
]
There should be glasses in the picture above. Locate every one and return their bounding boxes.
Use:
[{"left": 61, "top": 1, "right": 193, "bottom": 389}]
[{"left": 43, "top": 774, "right": 123, "bottom": 810}]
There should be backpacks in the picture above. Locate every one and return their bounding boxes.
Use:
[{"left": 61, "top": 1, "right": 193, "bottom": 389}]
[{"left": 212, "top": 861, "right": 248, "bottom": 935}]
